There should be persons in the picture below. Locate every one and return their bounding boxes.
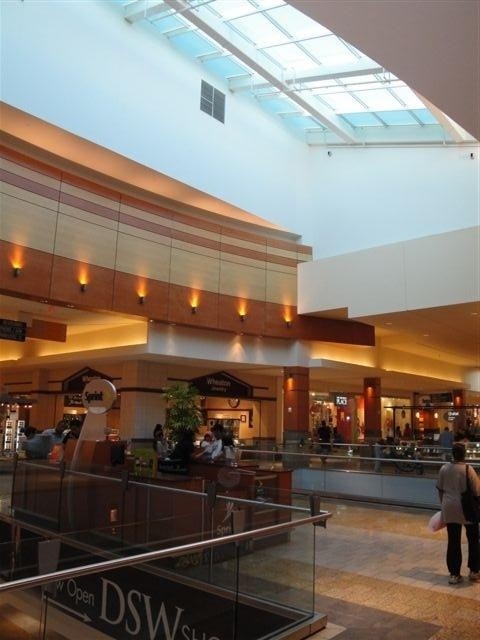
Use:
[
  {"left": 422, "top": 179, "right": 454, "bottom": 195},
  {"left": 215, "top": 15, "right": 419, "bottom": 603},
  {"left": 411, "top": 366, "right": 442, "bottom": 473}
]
[
  {"left": 440, "top": 427, "right": 465, "bottom": 446},
  {"left": 396, "top": 423, "right": 411, "bottom": 438},
  {"left": 318, "top": 421, "right": 331, "bottom": 449},
  {"left": 41, "top": 419, "right": 82, "bottom": 450},
  {"left": 153, "top": 424, "right": 163, "bottom": 451},
  {"left": 192, "top": 423, "right": 234, "bottom": 466},
  {"left": 437, "top": 442, "right": 480, "bottom": 584}
]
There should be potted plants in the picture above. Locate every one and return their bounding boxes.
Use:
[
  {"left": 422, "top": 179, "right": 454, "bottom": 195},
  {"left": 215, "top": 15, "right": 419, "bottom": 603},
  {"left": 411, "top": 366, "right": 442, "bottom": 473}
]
[{"left": 161, "top": 378, "right": 206, "bottom": 459}]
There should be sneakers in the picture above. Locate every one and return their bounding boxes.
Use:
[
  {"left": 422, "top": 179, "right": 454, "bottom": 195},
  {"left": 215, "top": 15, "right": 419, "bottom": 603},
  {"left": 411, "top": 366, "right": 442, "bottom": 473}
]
[
  {"left": 448, "top": 575, "right": 464, "bottom": 585},
  {"left": 469, "top": 572, "right": 480, "bottom": 583}
]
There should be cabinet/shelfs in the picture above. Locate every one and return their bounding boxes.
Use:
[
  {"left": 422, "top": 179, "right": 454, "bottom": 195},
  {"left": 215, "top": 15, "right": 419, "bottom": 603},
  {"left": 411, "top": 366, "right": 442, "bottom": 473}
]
[{"left": 10, "top": 459, "right": 294, "bottom": 568}]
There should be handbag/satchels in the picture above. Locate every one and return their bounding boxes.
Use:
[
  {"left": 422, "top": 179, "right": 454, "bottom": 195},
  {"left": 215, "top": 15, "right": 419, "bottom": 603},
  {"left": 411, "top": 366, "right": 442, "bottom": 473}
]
[
  {"left": 462, "top": 492, "right": 479, "bottom": 523},
  {"left": 213, "top": 453, "right": 225, "bottom": 467}
]
